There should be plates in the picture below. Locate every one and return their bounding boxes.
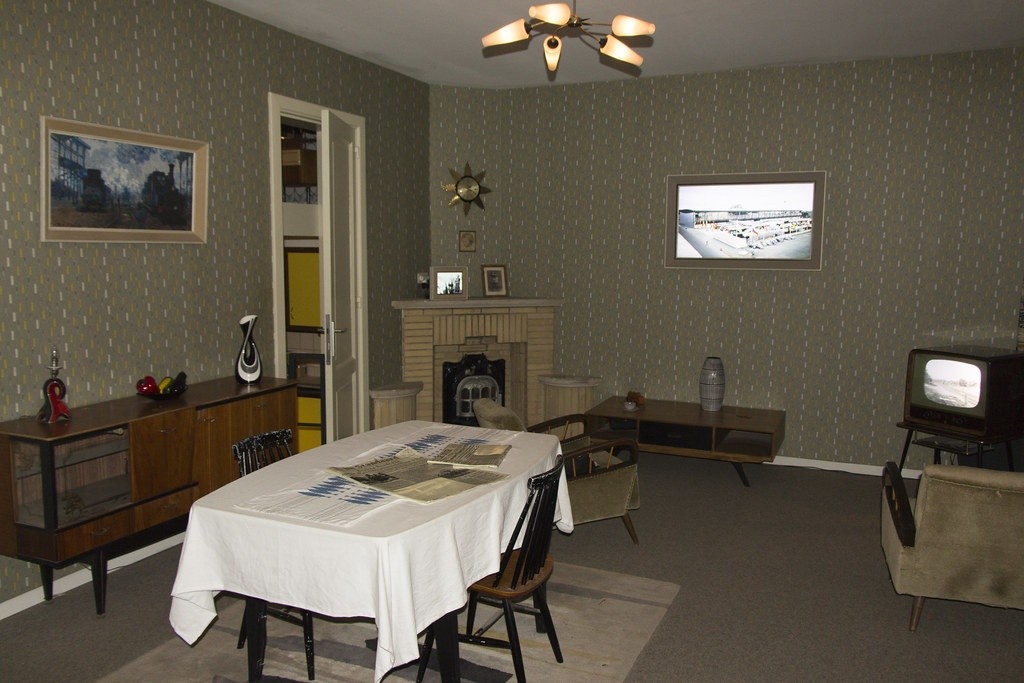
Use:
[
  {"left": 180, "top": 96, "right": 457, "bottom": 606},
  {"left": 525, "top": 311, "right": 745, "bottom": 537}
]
[{"left": 136, "top": 383, "right": 189, "bottom": 400}]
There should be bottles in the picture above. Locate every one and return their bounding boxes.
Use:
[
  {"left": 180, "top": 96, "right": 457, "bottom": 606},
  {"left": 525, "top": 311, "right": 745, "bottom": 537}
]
[{"left": 235, "top": 315, "right": 263, "bottom": 385}]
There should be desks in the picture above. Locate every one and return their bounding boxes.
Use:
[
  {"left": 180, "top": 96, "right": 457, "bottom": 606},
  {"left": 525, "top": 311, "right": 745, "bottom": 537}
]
[
  {"left": 169, "top": 420, "right": 577, "bottom": 683},
  {"left": 894, "top": 422, "right": 1024, "bottom": 472},
  {"left": 583, "top": 395, "right": 787, "bottom": 488}
]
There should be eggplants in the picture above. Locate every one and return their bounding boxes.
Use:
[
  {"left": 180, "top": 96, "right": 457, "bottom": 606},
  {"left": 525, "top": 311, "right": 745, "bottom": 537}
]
[{"left": 172, "top": 371, "right": 187, "bottom": 394}]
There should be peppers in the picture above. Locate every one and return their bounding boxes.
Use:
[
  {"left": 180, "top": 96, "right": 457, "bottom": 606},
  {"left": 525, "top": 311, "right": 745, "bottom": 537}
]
[{"left": 136, "top": 376, "right": 172, "bottom": 393}]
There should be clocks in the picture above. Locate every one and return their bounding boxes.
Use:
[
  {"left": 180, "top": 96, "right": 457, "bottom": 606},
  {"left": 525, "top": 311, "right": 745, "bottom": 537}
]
[{"left": 440, "top": 162, "right": 493, "bottom": 213}]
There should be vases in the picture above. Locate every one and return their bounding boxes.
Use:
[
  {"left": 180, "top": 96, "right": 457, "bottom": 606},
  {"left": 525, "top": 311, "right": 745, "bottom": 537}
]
[{"left": 235, "top": 314, "right": 262, "bottom": 387}]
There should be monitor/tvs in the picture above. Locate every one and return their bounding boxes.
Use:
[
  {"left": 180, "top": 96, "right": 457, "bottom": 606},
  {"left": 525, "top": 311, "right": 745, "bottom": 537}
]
[{"left": 902, "top": 343, "right": 1024, "bottom": 445}]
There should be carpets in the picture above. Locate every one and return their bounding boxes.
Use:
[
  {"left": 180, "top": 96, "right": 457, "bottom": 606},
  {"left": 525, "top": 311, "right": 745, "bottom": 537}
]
[{"left": 96, "top": 560, "right": 682, "bottom": 683}]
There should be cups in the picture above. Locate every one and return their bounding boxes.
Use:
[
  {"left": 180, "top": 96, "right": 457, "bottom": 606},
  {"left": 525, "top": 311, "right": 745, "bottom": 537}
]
[{"left": 625, "top": 402, "right": 636, "bottom": 409}]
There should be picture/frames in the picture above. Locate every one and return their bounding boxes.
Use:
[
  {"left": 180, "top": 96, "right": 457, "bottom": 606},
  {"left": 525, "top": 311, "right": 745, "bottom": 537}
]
[
  {"left": 39, "top": 115, "right": 210, "bottom": 245},
  {"left": 479, "top": 264, "right": 508, "bottom": 298},
  {"left": 459, "top": 229, "right": 476, "bottom": 252},
  {"left": 429, "top": 266, "right": 467, "bottom": 301}
]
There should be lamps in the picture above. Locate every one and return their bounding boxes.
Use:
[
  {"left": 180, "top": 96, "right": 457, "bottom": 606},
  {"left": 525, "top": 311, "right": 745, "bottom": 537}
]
[{"left": 481, "top": 0, "right": 655, "bottom": 73}]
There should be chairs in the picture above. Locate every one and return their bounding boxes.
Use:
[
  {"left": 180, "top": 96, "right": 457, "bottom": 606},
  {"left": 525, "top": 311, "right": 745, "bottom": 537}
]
[
  {"left": 879, "top": 463, "right": 1023, "bottom": 634},
  {"left": 228, "top": 429, "right": 316, "bottom": 682},
  {"left": 415, "top": 454, "right": 563, "bottom": 683},
  {"left": 472, "top": 398, "right": 642, "bottom": 544}
]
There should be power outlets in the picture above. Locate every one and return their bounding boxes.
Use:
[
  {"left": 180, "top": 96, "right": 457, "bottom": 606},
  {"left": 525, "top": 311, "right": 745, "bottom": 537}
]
[{"left": 416, "top": 272, "right": 429, "bottom": 283}]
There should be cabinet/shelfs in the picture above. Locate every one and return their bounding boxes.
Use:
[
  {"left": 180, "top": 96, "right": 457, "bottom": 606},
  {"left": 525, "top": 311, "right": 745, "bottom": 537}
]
[
  {"left": 284, "top": 247, "right": 319, "bottom": 333},
  {"left": 0, "top": 376, "right": 300, "bottom": 619},
  {"left": 297, "top": 387, "right": 322, "bottom": 453}
]
